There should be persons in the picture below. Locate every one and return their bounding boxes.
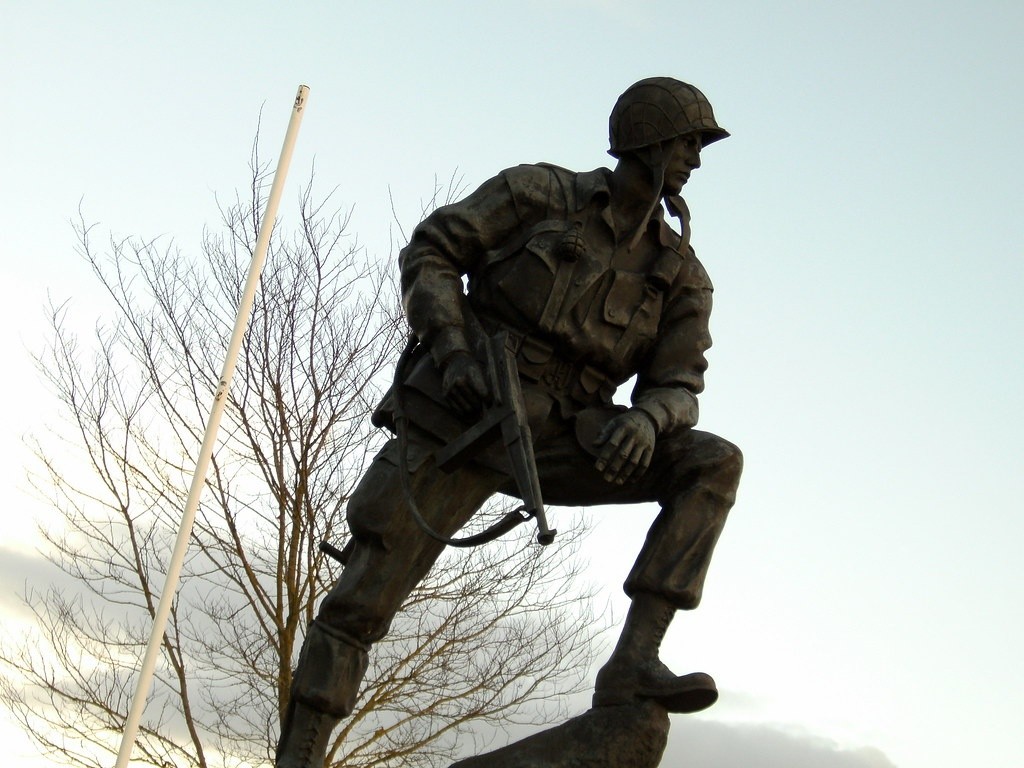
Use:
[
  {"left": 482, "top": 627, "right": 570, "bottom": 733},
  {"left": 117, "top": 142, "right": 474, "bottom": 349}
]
[{"left": 273, "top": 78, "right": 746, "bottom": 768}]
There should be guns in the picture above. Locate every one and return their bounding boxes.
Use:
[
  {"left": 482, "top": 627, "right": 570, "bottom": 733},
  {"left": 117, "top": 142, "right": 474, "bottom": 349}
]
[{"left": 431, "top": 294, "right": 556, "bottom": 545}]
